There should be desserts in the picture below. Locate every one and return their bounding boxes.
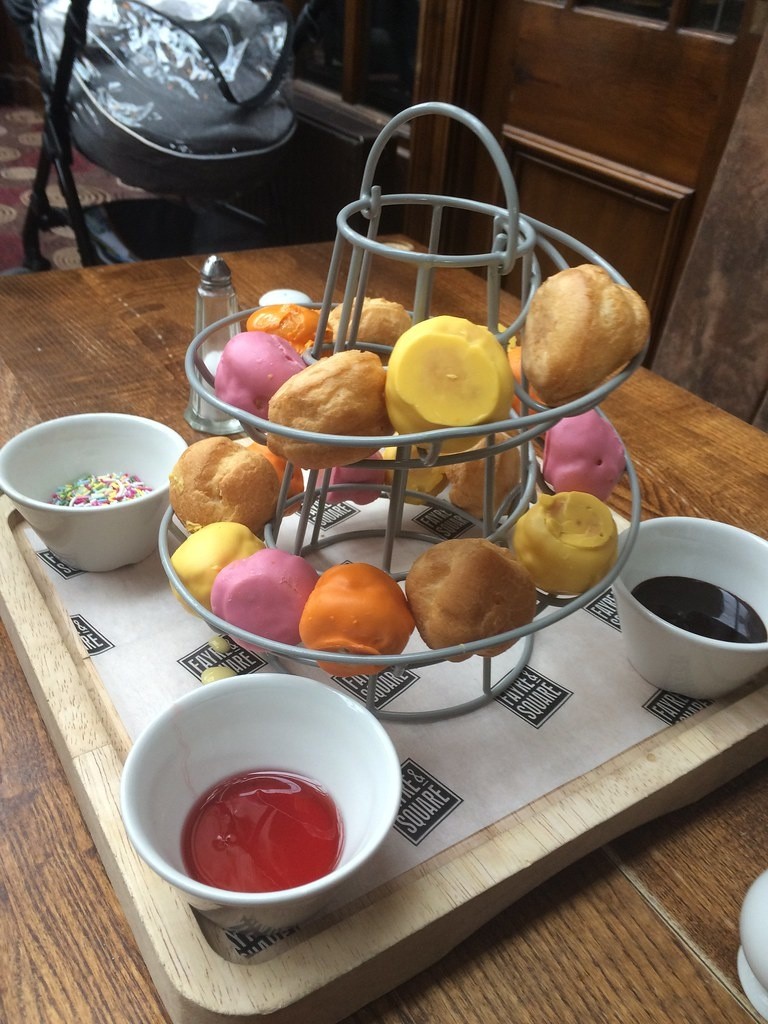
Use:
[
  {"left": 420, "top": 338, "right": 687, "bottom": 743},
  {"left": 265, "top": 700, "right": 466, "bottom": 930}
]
[{"left": 171, "top": 303, "right": 623, "bottom": 675}]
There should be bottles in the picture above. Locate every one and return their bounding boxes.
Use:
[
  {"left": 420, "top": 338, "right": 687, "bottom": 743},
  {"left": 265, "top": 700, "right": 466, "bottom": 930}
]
[{"left": 185, "top": 255, "right": 245, "bottom": 435}]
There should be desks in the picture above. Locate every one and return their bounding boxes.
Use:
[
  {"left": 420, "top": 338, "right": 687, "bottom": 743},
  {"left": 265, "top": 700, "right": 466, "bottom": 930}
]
[{"left": 1, "top": 231, "right": 767, "bottom": 1024}]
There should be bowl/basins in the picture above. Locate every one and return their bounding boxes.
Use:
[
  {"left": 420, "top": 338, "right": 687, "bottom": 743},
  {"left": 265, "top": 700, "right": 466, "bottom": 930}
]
[
  {"left": 119, "top": 673, "right": 403, "bottom": 937},
  {"left": 0, "top": 412, "right": 189, "bottom": 572},
  {"left": 612, "top": 515, "right": 768, "bottom": 700}
]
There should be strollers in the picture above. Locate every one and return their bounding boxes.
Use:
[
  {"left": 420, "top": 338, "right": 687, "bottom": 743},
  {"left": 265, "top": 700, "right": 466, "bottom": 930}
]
[{"left": 0, "top": 0, "right": 326, "bottom": 281}]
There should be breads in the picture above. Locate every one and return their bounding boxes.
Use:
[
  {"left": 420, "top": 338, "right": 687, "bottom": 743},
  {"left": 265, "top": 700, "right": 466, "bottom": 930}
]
[
  {"left": 445, "top": 430, "right": 522, "bottom": 521},
  {"left": 327, "top": 296, "right": 412, "bottom": 366},
  {"left": 169, "top": 436, "right": 282, "bottom": 537},
  {"left": 265, "top": 348, "right": 396, "bottom": 469},
  {"left": 405, "top": 537, "right": 536, "bottom": 663},
  {"left": 522, "top": 264, "right": 649, "bottom": 408}
]
